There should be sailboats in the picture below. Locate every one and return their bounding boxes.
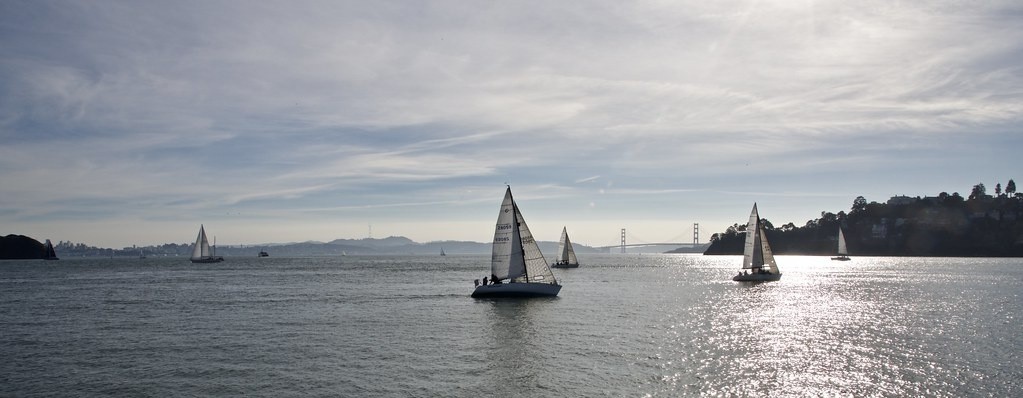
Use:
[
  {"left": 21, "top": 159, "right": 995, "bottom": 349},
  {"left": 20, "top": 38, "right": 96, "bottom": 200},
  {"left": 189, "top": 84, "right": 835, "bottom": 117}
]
[
  {"left": 832, "top": 228, "right": 849, "bottom": 262},
  {"left": 471, "top": 187, "right": 562, "bottom": 297},
  {"left": 552, "top": 226, "right": 580, "bottom": 268},
  {"left": 733, "top": 200, "right": 782, "bottom": 284},
  {"left": 189, "top": 224, "right": 221, "bottom": 263}
]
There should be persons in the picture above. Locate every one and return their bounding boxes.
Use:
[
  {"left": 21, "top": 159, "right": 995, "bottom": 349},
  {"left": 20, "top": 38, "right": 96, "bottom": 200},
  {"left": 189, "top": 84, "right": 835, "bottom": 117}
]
[{"left": 483, "top": 274, "right": 501, "bottom": 286}]
